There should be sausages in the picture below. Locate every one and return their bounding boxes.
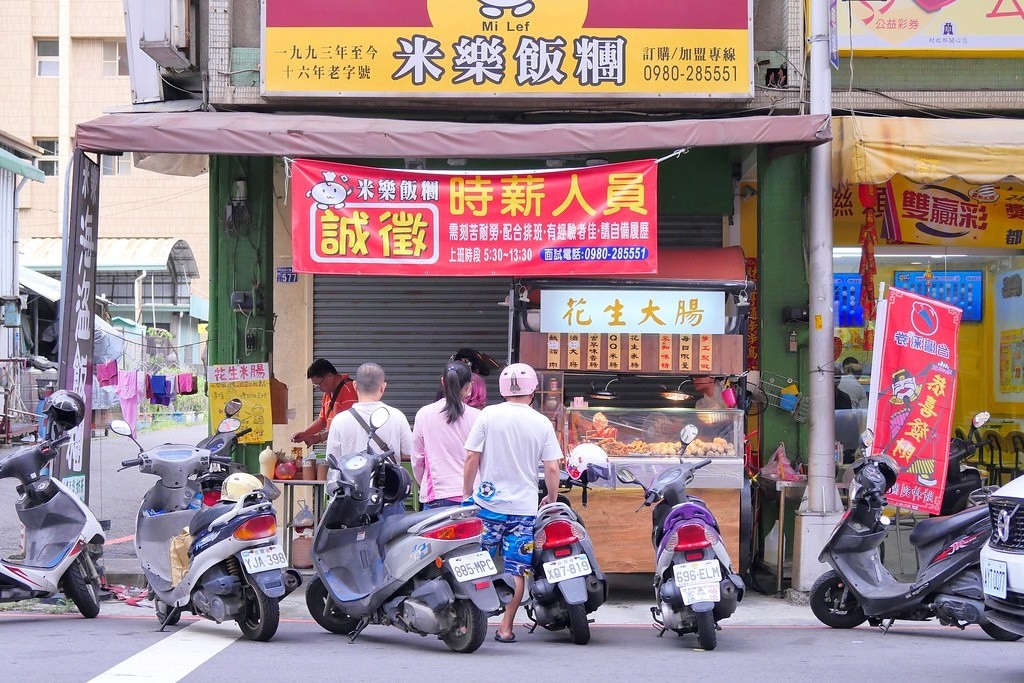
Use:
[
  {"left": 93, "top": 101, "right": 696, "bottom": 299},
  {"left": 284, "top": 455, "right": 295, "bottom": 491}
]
[{"left": 597, "top": 427, "right": 617, "bottom": 437}]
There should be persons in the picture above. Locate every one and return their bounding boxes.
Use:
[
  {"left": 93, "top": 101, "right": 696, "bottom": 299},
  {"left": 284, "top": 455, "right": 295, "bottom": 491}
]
[
  {"left": 326, "top": 360, "right": 416, "bottom": 562},
  {"left": 690, "top": 375, "right": 737, "bottom": 445},
  {"left": 411, "top": 361, "right": 483, "bottom": 511},
  {"left": 461, "top": 362, "right": 564, "bottom": 644},
  {"left": 833, "top": 357, "right": 868, "bottom": 465},
  {"left": 290, "top": 358, "right": 358, "bottom": 450},
  {"left": 452, "top": 347, "right": 491, "bottom": 410}
]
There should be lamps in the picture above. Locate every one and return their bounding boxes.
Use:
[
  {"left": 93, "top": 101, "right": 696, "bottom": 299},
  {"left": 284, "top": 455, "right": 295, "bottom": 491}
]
[
  {"left": 661, "top": 379, "right": 692, "bottom": 402},
  {"left": 586, "top": 381, "right": 619, "bottom": 399}
]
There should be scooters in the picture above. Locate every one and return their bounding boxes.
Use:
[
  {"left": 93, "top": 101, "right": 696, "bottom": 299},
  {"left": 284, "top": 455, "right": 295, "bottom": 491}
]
[
  {"left": 306, "top": 406, "right": 504, "bottom": 653},
  {"left": 809, "top": 427, "right": 993, "bottom": 635},
  {"left": 929, "top": 410, "right": 1001, "bottom": 516},
  {"left": 0, "top": 390, "right": 112, "bottom": 619},
  {"left": 507, "top": 442, "right": 615, "bottom": 645},
  {"left": 615, "top": 422, "right": 746, "bottom": 651},
  {"left": 110, "top": 398, "right": 304, "bottom": 641}
]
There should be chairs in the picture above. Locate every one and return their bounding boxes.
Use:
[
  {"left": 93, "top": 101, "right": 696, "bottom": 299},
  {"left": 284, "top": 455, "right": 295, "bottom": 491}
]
[{"left": 955, "top": 424, "right": 1023, "bottom": 486}]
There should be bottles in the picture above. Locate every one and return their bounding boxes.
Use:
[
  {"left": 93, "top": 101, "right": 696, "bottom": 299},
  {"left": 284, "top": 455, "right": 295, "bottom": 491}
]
[
  {"left": 316, "top": 454, "right": 326, "bottom": 469},
  {"left": 549, "top": 377, "right": 558, "bottom": 391},
  {"left": 296, "top": 456, "right": 303, "bottom": 471},
  {"left": 550, "top": 418, "right": 556, "bottom": 430},
  {"left": 294, "top": 499, "right": 314, "bottom": 533},
  {"left": 764, "top": 519, "right": 786, "bottom": 564},
  {"left": 188, "top": 493, "right": 202, "bottom": 509},
  {"left": 876, "top": 515, "right": 890, "bottom": 533},
  {"left": 292, "top": 535, "right": 313, "bottom": 568},
  {"left": 259, "top": 446, "right": 277, "bottom": 480},
  {"left": 546, "top": 397, "right": 557, "bottom": 411}
]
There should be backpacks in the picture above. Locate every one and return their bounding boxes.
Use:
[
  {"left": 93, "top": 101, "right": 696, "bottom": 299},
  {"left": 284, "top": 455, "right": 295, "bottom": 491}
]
[{"left": 170, "top": 526, "right": 195, "bottom": 588}]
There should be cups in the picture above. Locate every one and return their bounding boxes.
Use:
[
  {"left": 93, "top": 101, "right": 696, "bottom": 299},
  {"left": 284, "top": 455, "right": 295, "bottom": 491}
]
[{"left": 721, "top": 386, "right": 737, "bottom": 408}]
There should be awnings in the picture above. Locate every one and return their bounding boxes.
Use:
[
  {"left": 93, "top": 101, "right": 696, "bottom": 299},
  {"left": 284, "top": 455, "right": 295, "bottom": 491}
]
[{"left": 831, "top": 115, "right": 1024, "bottom": 185}]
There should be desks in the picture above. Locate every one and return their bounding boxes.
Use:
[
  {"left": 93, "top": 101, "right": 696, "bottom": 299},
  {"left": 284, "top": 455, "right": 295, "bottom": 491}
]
[{"left": 270, "top": 479, "right": 329, "bottom": 574}]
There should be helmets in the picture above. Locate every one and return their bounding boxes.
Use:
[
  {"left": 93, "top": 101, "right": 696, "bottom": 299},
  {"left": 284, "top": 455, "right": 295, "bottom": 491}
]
[
  {"left": 565, "top": 443, "right": 611, "bottom": 481},
  {"left": 853, "top": 454, "right": 900, "bottom": 488},
  {"left": 42, "top": 389, "right": 85, "bottom": 432},
  {"left": 498, "top": 362, "right": 539, "bottom": 397},
  {"left": 216, "top": 472, "right": 265, "bottom": 502},
  {"left": 379, "top": 461, "right": 412, "bottom": 504}
]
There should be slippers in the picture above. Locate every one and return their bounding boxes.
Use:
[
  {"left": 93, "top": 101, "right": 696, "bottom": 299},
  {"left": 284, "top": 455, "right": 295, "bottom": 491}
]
[{"left": 493, "top": 628, "right": 518, "bottom": 643}]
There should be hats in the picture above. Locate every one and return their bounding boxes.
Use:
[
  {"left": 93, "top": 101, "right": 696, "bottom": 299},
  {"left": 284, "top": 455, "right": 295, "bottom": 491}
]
[{"left": 456, "top": 347, "right": 492, "bottom": 377}]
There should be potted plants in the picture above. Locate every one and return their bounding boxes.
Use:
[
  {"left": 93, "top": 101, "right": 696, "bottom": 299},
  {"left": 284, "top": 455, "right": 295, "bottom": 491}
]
[{"left": 109, "top": 328, "right": 207, "bottom": 426}]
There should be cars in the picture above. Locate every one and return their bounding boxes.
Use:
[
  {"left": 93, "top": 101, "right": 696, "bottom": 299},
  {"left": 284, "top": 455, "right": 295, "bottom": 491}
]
[{"left": 978, "top": 475, "right": 1024, "bottom": 637}]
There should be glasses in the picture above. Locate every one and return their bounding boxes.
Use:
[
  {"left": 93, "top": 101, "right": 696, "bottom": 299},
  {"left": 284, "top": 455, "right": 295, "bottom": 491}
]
[{"left": 313, "top": 374, "right": 329, "bottom": 389}]
